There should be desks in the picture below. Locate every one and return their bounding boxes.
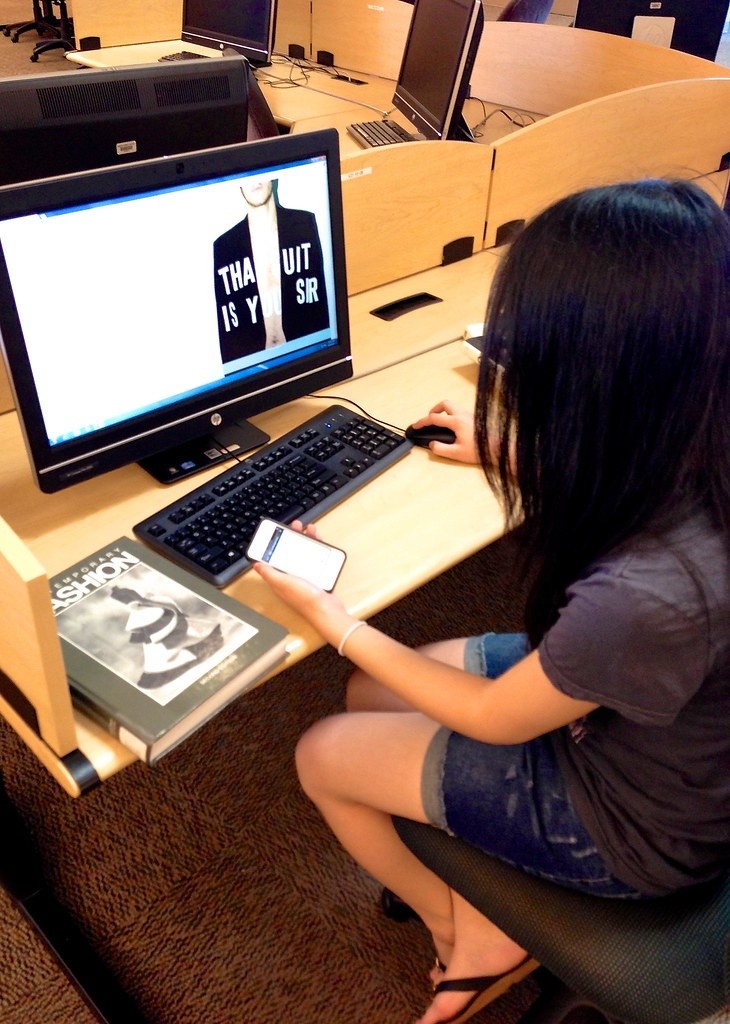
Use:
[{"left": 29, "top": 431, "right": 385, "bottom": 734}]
[{"left": 0, "top": 0, "right": 730, "bottom": 1024}]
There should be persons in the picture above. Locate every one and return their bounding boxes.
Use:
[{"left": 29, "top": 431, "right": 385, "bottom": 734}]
[
  {"left": 248, "top": 180, "right": 730, "bottom": 1024},
  {"left": 213, "top": 178, "right": 331, "bottom": 365}
]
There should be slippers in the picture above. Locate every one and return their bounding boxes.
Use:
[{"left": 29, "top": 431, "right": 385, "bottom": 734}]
[{"left": 432, "top": 956, "right": 540, "bottom": 1024}]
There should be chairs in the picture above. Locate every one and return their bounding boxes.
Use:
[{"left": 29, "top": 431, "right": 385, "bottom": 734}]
[
  {"left": 221, "top": 45, "right": 277, "bottom": 141},
  {"left": 382, "top": 812, "right": 729, "bottom": 1023}
]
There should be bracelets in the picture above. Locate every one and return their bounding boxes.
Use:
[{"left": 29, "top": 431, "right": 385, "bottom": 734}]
[{"left": 337, "top": 621, "right": 367, "bottom": 657}]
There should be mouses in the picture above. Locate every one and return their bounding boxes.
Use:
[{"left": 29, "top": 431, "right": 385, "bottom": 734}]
[{"left": 405, "top": 425, "right": 456, "bottom": 449}]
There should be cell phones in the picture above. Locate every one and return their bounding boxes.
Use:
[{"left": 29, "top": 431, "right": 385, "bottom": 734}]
[{"left": 245, "top": 517, "right": 347, "bottom": 593}]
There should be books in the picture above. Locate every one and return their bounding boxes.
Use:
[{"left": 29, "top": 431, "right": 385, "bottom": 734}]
[{"left": 45, "top": 535, "right": 302, "bottom": 766}]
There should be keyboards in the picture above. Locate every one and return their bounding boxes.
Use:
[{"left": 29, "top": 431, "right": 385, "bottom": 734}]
[
  {"left": 158, "top": 51, "right": 211, "bottom": 62},
  {"left": 347, "top": 120, "right": 418, "bottom": 149},
  {"left": 131, "top": 404, "right": 414, "bottom": 588}
]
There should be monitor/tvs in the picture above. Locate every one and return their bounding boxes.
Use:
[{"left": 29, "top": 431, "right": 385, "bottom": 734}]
[
  {"left": 0, "top": 55, "right": 354, "bottom": 495},
  {"left": 572, "top": 0, "right": 730, "bottom": 62},
  {"left": 392, "top": 0, "right": 485, "bottom": 143},
  {"left": 181, "top": 0, "right": 278, "bottom": 71}
]
